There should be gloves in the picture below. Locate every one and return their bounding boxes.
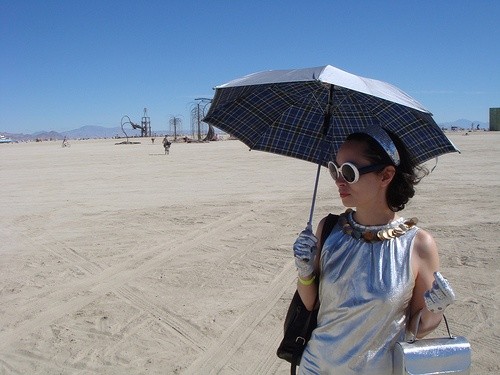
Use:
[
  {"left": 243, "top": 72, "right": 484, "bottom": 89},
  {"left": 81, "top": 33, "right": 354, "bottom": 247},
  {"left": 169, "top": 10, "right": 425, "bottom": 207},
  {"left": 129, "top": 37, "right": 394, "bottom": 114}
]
[
  {"left": 423, "top": 271, "right": 455, "bottom": 313},
  {"left": 292, "top": 230, "right": 319, "bottom": 278}
]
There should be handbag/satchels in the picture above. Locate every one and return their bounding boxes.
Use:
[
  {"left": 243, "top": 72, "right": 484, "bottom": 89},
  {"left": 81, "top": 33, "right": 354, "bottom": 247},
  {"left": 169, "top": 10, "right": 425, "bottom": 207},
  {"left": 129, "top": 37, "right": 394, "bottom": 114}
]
[
  {"left": 277, "top": 213, "right": 339, "bottom": 375},
  {"left": 393, "top": 308, "right": 472, "bottom": 375}
]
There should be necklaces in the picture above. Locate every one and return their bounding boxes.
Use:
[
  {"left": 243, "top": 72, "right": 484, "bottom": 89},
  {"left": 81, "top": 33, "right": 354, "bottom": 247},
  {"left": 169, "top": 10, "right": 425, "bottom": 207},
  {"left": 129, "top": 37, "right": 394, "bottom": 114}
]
[{"left": 340, "top": 208, "right": 418, "bottom": 241}]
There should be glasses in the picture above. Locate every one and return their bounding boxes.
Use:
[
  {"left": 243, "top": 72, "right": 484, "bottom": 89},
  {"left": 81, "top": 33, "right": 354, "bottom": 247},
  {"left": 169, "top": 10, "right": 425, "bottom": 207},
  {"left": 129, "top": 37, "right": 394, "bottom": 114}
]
[{"left": 328, "top": 160, "right": 386, "bottom": 184}]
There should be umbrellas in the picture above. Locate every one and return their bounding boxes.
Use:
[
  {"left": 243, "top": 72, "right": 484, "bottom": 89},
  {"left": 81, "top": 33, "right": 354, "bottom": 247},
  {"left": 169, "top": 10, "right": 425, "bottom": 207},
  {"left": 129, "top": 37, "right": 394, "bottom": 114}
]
[{"left": 199, "top": 64, "right": 461, "bottom": 264}]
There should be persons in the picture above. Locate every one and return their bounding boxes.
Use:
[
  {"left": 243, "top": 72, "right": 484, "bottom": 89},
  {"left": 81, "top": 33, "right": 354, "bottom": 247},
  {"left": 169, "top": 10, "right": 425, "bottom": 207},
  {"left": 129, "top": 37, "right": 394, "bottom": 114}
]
[
  {"left": 293, "top": 128, "right": 455, "bottom": 375},
  {"left": 162, "top": 134, "right": 170, "bottom": 152}
]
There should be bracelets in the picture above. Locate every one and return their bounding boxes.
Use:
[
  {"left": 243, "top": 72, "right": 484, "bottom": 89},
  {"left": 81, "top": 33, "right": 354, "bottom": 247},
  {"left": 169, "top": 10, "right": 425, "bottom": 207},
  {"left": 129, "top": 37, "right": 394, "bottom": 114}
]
[{"left": 298, "top": 276, "right": 316, "bottom": 284}]
